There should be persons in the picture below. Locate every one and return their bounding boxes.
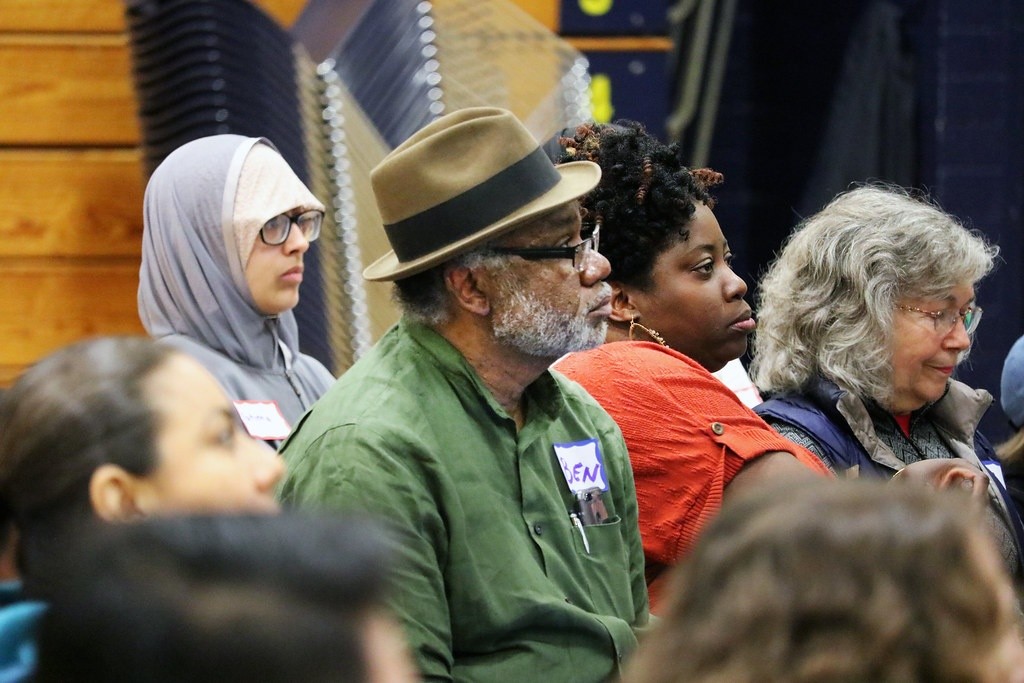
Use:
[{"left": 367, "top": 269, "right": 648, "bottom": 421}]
[{"left": 0, "top": 108, "right": 1024, "bottom": 683}]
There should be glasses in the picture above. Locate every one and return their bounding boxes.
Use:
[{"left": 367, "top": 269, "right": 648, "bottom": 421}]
[
  {"left": 894, "top": 303, "right": 982, "bottom": 335},
  {"left": 477, "top": 221, "right": 600, "bottom": 273},
  {"left": 259, "top": 210, "right": 325, "bottom": 246}
]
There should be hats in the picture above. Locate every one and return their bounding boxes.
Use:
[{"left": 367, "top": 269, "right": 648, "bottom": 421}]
[
  {"left": 232, "top": 143, "right": 329, "bottom": 272},
  {"left": 362, "top": 107, "right": 603, "bottom": 281},
  {"left": 1001, "top": 335, "right": 1024, "bottom": 432}
]
